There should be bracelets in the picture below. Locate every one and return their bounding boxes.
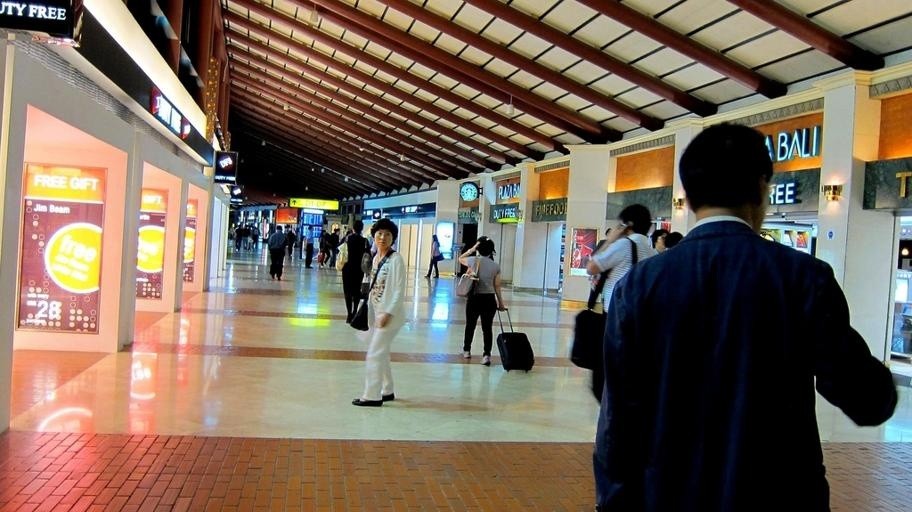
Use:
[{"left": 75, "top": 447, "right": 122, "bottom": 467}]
[{"left": 467, "top": 248, "right": 472, "bottom": 255}]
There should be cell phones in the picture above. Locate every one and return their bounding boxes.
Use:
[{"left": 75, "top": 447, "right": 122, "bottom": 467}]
[{"left": 620, "top": 224, "right": 628, "bottom": 230}]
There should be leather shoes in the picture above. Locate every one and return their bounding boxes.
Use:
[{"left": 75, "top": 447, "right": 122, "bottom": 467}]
[
  {"left": 382, "top": 393, "right": 394, "bottom": 401},
  {"left": 352, "top": 398, "right": 383, "bottom": 406}
]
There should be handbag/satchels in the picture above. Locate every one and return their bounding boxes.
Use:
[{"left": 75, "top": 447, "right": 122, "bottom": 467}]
[
  {"left": 350, "top": 303, "right": 369, "bottom": 330},
  {"left": 437, "top": 253, "right": 444, "bottom": 261},
  {"left": 318, "top": 255, "right": 324, "bottom": 262},
  {"left": 571, "top": 309, "right": 607, "bottom": 371},
  {"left": 335, "top": 241, "right": 349, "bottom": 271},
  {"left": 361, "top": 253, "right": 373, "bottom": 274},
  {"left": 456, "top": 258, "right": 480, "bottom": 295}
]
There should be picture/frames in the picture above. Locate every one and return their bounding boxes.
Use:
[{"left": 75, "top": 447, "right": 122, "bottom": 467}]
[{"left": 569, "top": 227, "right": 600, "bottom": 277}]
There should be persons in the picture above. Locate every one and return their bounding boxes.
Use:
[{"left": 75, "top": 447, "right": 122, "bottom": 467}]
[
  {"left": 351, "top": 219, "right": 406, "bottom": 407},
  {"left": 423, "top": 234, "right": 442, "bottom": 278},
  {"left": 334, "top": 220, "right": 371, "bottom": 324},
  {"left": 457, "top": 235, "right": 504, "bottom": 366},
  {"left": 592, "top": 121, "right": 899, "bottom": 511},
  {"left": 585, "top": 203, "right": 681, "bottom": 406},
  {"left": 235, "top": 223, "right": 394, "bottom": 281}
]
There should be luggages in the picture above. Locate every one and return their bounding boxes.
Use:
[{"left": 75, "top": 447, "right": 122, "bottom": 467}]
[{"left": 497, "top": 307, "right": 533, "bottom": 373}]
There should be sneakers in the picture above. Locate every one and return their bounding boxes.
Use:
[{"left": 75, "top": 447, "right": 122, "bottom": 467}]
[
  {"left": 463, "top": 351, "right": 471, "bottom": 358},
  {"left": 424, "top": 275, "right": 439, "bottom": 279},
  {"left": 482, "top": 355, "right": 491, "bottom": 366}
]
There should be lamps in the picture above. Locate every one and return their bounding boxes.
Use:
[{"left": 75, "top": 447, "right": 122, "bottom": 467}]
[
  {"left": 506, "top": 95, "right": 513, "bottom": 115},
  {"left": 821, "top": 185, "right": 843, "bottom": 202},
  {"left": 311, "top": 4, "right": 318, "bottom": 22},
  {"left": 673, "top": 198, "right": 685, "bottom": 209}
]
